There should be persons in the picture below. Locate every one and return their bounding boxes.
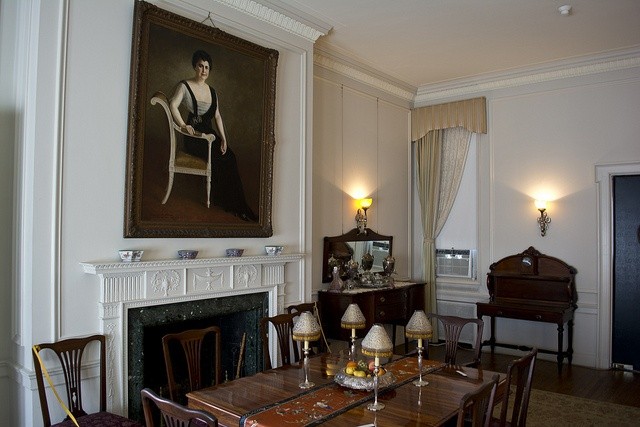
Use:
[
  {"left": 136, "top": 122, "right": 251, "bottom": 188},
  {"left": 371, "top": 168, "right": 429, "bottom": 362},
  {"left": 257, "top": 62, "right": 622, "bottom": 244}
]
[{"left": 170, "top": 50, "right": 257, "bottom": 222}]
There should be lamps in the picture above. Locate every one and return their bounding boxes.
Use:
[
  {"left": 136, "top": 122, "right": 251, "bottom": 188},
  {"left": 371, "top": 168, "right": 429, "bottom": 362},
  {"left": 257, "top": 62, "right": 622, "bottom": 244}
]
[
  {"left": 557, "top": 4, "right": 571, "bottom": 18},
  {"left": 406, "top": 309, "right": 433, "bottom": 386},
  {"left": 340, "top": 304, "right": 366, "bottom": 360},
  {"left": 292, "top": 310, "right": 321, "bottom": 389},
  {"left": 360, "top": 195, "right": 372, "bottom": 227},
  {"left": 534, "top": 190, "right": 550, "bottom": 233},
  {"left": 363, "top": 324, "right": 393, "bottom": 412}
]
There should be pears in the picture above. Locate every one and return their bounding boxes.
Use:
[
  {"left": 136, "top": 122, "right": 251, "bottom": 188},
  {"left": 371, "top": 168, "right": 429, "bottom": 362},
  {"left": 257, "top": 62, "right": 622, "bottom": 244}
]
[
  {"left": 353, "top": 370, "right": 366, "bottom": 378},
  {"left": 346, "top": 367, "right": 356, "bottom": 374}
]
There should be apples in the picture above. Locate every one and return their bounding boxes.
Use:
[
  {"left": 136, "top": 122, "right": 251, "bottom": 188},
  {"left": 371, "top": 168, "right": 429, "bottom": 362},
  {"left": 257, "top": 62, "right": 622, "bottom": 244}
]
[
  {"left": 373, "top": 367, "right": 385, "bottom": 377},
  {"left": 368, "top": 360, "right": 375, "bottom": 370}
]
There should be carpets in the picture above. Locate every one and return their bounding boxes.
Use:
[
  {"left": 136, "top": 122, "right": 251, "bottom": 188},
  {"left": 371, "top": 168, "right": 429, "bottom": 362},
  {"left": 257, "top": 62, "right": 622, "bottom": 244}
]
[{"left": 494, "top": 384, "right": 640, "bottom": 427}]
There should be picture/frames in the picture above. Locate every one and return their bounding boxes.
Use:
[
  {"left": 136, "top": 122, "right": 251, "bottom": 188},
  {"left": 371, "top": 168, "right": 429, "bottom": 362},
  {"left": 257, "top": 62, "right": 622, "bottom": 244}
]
[{"left": 124, "top": 0, "right": 279, "bottom": 238}]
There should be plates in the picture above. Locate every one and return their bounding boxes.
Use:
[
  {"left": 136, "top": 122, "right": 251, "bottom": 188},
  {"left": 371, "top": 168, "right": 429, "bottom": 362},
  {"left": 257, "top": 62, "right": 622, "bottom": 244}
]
[{"left": 334, "top": 363, "right": 397, "bottom": 391}]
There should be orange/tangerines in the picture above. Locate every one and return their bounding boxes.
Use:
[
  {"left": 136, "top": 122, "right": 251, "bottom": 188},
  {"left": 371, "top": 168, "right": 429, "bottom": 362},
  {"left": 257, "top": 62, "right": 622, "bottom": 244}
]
[{"left": 344, "top": 361, "right": 357, "bottom": 367}]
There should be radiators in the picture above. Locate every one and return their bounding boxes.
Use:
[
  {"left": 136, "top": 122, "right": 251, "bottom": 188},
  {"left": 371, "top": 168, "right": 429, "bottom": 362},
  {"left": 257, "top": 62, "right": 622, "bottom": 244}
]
[{"left": 435, "top": 247, "right": 476, "bottom": 279}]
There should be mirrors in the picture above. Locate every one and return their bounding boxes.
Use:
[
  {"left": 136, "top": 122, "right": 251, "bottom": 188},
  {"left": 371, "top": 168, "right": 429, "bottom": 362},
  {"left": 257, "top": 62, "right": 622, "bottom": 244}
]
[{"left": 322, "top": 228, "right": 393, "bottom": 283}]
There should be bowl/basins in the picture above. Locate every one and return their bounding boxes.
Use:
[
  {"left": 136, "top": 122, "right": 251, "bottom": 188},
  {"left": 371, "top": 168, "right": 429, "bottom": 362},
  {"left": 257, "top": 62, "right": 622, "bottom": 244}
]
[
  {"left": 118, "top": 250, "right": 144, "bottom": 264},
  {"left": 265, "top": 245, "right": 285, "bottom": 256},
  {"left": 178, "top": 249, "right": 198, "bottom": 258},
  {"left": 225, "top": 249, "right": 245, "bottom": 257}
]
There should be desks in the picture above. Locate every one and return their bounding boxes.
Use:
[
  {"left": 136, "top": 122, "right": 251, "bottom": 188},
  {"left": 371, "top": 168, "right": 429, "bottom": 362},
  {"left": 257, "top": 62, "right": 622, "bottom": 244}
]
[
  {"left": 317, "top": 281, "right": 427, "bottom": 354},
  {"left": 476, "top": 298, "right": 578, "bottom": 376}
]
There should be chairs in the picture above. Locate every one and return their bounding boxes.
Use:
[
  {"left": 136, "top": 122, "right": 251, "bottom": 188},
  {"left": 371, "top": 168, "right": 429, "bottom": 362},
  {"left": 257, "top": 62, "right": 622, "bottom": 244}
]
[
  {"left": 161, "top": 326, "right": 223, "bottom": 405},
  {"left": 424, "top": 313, "right": 483, "bottom": 367},
  {"left": 140, "top": 388, "right": 218, "bottom": 427},
  {"left": 491, "top": 347, "right": 538, "bottom": 426},
  {"left": 150, "top": 89, "right": 216, "bottom": 209},
  {"left": 260, "top": 312, "right": 300, "bottom": 370},
  {"left": 288, "top": 301, "right": 326, "bottom": 363},
  {"left": 456, "top": 374, "right": 500, "bottom": 426},
  {"left": 32, "top": 335, "right": 145, "bottom": 427}
]
